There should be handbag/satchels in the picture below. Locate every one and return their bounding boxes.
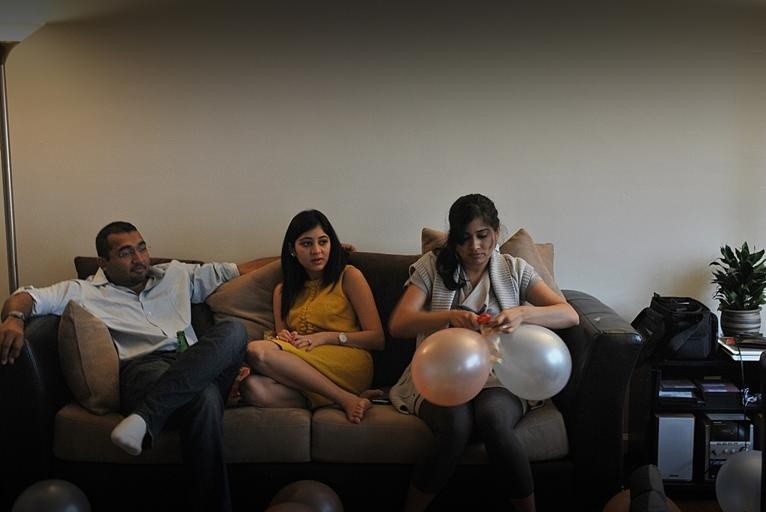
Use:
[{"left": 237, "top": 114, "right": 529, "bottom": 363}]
[{"left": 632, "top": 292, "right": 719, "bottom": 380}]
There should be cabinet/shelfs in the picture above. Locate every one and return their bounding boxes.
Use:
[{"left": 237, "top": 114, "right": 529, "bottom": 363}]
[{"left": 637, "top": 338, "right": 765, "bottom": 501}]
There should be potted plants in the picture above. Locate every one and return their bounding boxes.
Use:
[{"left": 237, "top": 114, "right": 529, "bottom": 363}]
[{"left": 709, "top": 239, "right": 766, "bottom": 338}]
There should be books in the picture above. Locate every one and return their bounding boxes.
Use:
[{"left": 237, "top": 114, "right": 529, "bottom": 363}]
[
  {"left": 658, "top": 373, "right": 740, "bottom": 404},
  {"left": 718, "top": 335, "right": 765, "bottom": 362}
]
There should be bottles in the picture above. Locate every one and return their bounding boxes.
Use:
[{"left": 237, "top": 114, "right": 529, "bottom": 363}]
[{"left": 175, "top": 331, "right": 190, "bottom": 361}]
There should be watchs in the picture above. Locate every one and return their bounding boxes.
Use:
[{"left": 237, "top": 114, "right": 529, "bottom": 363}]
[
  {"left": 2, "top": 310, "right": 26, "bottom": 325},
  {"left": 337, "top": 332, "right": 347, "bottom": 345}
]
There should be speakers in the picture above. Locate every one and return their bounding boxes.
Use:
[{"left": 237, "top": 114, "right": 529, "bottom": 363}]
[{"left": 653, "top": 412, "right": 695, "bottom": 483}]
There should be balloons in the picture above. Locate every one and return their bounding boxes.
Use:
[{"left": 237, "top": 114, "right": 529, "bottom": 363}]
[
  {"left": 13, "top": 478, "right": 91, "bottom": 512},
  {"left": 273, "top": 479, "right": 342, "bottom": 512},
  {"left": 492, "top": 322, "right": 572, "bottom": 399},
  {"left": 263, "top": 501, "right": 317, "bottom": 512},
  {"left": 411, "top": 327, "right": 492, "bottom": 407}
]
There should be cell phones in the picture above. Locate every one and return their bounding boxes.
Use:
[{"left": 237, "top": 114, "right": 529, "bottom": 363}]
[{"left": 371, "top": 398, "right": 390, "bottom": 403}]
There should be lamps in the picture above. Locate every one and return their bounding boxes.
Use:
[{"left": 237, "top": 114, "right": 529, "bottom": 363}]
[{"left": 0, "top": 19, "right": 50, "bottom": 295}]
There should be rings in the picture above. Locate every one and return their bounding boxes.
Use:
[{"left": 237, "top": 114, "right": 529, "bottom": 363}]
[
  {"left": 501, "top": 323, "right": 510, "bottom": 328},
  {"left": 307, "top": 339, "right": 312, "bottom": 344}
]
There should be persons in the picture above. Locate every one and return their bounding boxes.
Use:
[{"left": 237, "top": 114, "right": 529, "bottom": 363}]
[
  {"left": 0, "top": 220, "right": 355, "bottom": 510},
  {"left": 388, "top": 194, "right": 580, "bottom": 510},
  {"left": 238, "top": 210, "right": 386, "bottom": 424}
]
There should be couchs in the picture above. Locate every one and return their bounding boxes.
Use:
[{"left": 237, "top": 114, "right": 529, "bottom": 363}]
[{"left": 1, "top": 253, "right": 642, "bottom": 509}]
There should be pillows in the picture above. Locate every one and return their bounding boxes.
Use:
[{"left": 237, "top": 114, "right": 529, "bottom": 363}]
[
  {"left": 204, "top": 260, "right": 283, "bottom": 350},
  {"left": 51, "top": 298, "right": 120, "bottom": 418},
  {"left": 421, "top": 226, "right": 552, "bottom": 275}
]
